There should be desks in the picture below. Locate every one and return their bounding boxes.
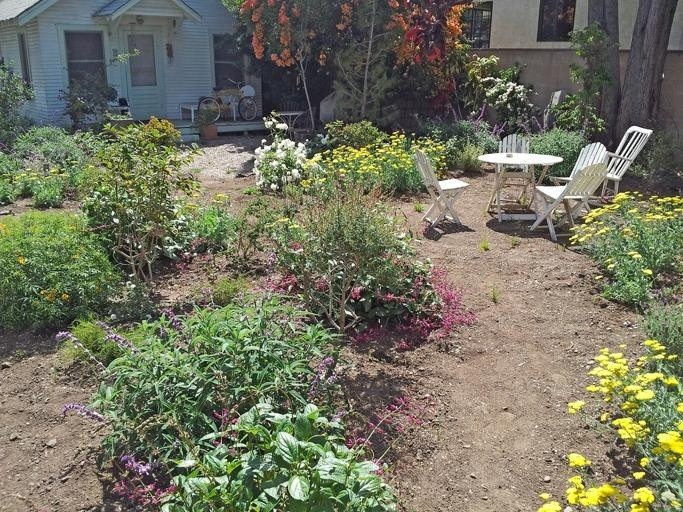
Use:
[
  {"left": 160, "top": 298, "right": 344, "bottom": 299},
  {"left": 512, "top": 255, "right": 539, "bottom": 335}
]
[
  {"left": 178, "top": 103, "right": 235, "bottom": 122},
  {"left": 477, "top": 153, "right": 563, "bottom": 221}
]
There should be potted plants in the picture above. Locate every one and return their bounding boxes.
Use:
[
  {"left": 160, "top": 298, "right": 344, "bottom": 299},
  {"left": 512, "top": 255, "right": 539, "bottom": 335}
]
[
  {"left": 107, "top": 113, "right": 132, "bottom": 128},
  {"left": 196, "top": 108, "right": 217, "bottom": 138}
]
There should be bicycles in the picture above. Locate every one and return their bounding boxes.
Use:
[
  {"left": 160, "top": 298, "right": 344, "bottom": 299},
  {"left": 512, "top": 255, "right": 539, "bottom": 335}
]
[{"left": 196, "top": 77, "right": 258, "bottom": 123}]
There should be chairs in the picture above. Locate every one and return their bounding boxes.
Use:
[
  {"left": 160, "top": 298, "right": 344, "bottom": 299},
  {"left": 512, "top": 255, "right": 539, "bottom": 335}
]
[
  {"left": 529, "top": 162, "right": 607, "bottom": 244},
  {"left": 273, "top": 99, "right": 321, "bottom": 144},
  {"left": 549, "top": 141, "right": 608, "bottom": 219},
  {"left": 497, "top": 133, "right": 530, "bottom": 201},
  {"left": 600, "top": 125, "right": 652, "bottom": 198},
  {"left": 415, "top": 150, "right": 468, "bottom": 228}
]
